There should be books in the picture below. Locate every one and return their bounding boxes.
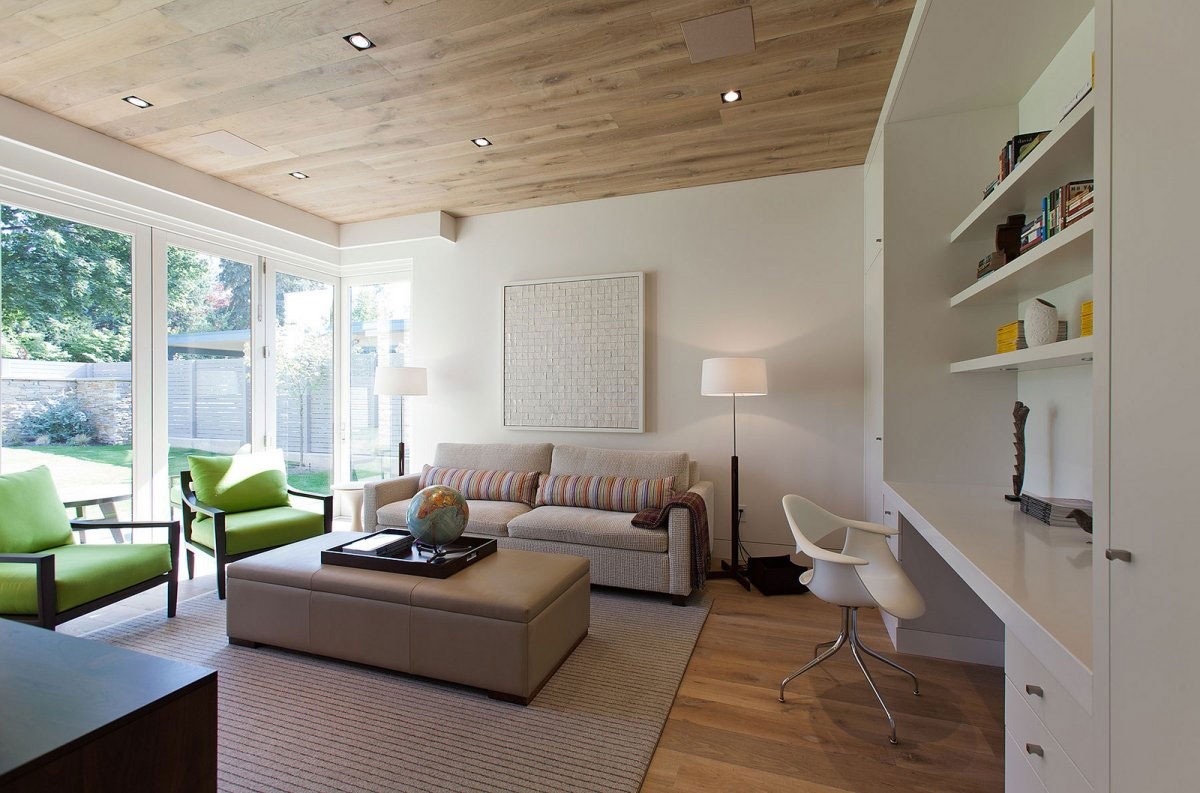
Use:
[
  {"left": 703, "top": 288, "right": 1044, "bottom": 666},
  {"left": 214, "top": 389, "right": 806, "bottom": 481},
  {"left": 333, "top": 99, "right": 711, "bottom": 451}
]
[
  {"left": 977, "top": 252, "right": 1006, "bottom": 279},
  {"left": 1042, "top": 180, "right": 1095, "bottom": 243},
  {"left": 1056, "top": 50, "right": 1095, "bottom": 123},
  {"left": 983, "top": 129, "right": 1052, "bottom": 200},
  {"left": 1080, "top": 301, "right": 1094, "bottom": 338},
  {"left": 995, "top": 319, "right": 1067, "bottom": 354},
  {"left": 1020, "top": 214, "right": 1044, "bottom": 255}
]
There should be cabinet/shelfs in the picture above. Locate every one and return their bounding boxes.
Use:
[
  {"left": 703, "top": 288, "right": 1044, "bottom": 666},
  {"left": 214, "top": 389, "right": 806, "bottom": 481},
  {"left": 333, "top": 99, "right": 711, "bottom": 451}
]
[
  {"left": 882, "top": 498, "right": 1005, "bottom": 666},
  {"left": 949, "top": 82, "right": 1093, "bottom": 530},
  {"left": 864, "top": 97, "right": 1019, "bottom": 530},
  {"left": 1004, "top": 625, "right": 1096, "bottom": 793}
]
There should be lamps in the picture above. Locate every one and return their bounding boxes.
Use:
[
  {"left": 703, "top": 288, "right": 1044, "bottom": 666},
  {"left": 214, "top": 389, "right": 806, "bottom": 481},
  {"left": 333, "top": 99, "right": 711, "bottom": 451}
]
[
  {"left": 374, "top": 366, "right": 427, "bottom": 476},
  {"left": 700, "top": 358, "right": 768, "bottom": 591}
]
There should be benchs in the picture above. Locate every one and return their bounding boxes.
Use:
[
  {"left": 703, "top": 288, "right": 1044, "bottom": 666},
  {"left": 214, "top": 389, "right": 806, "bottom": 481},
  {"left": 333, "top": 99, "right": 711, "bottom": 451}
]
[{"left": 227, "top": 530, "right": 591, "bottom": 706}]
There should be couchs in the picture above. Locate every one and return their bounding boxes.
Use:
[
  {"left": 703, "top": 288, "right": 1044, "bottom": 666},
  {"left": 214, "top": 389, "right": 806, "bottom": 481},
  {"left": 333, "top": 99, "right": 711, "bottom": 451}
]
[{"left": 362, "top": 443, "right": 715, "bottom": 607}]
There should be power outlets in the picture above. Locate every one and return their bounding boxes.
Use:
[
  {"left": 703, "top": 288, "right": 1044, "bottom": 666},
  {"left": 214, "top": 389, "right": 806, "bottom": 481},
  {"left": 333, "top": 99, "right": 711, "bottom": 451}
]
[{"left": 739, "top": 505, "right": 748, "bottom": 522}]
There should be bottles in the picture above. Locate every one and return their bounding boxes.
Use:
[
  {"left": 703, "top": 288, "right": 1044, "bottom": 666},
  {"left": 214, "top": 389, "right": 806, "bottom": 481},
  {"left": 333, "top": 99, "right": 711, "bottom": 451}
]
[{"left": 1023, "top": 298, "right": 1059, "bottom": 348}]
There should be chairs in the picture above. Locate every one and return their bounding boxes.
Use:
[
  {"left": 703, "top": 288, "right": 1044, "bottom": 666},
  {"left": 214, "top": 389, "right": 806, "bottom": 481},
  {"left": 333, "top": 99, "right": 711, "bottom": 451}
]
[
  {"left": 778, "top": 493, "right": 920, "bottom": 744},
  {"left": 180, "top": 470, "right": 333, "bottom": 600},
  {"left": 0, "top": 517, "right": 180, "bottom": 632}
]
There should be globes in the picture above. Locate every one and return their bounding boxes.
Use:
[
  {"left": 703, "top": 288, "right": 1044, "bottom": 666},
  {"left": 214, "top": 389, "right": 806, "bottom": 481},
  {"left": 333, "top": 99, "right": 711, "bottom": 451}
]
[{"left": 405, "top": 485, "right": 473, "bottom": 563}]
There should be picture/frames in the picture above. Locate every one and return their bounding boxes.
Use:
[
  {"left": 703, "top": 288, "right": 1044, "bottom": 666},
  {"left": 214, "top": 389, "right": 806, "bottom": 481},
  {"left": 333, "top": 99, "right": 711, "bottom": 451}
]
[{"left": 500, "top": 271, "right": 646, "bottom": 433}]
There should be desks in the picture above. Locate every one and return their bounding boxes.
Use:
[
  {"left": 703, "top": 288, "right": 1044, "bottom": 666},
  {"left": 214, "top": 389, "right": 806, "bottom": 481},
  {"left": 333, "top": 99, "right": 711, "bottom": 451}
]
[{"left": 0, "top": 617, "right": 219, "bottom": 793}]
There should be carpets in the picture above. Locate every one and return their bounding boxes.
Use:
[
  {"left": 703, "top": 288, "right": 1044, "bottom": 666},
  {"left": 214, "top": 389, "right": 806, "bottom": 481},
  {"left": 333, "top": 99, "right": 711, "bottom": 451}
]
[{"left": 73, "top": 588, "right": 715, "bottom": 793}]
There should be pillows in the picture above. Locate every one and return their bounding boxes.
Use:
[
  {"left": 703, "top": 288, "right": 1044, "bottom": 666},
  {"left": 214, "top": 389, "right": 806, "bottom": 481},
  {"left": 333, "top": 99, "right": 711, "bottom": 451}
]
[
  {"left": 0, "top": 464, "right": 75, "bottom": 554},
  {"left": 535, "top": 472, "right": 677, "bottom": 512},
  {"left": 417, "top": 463, "right": 542, "bottom": 509},
  {"left": 186, "top": 448, "right": 292, "bottom": 523}
]
